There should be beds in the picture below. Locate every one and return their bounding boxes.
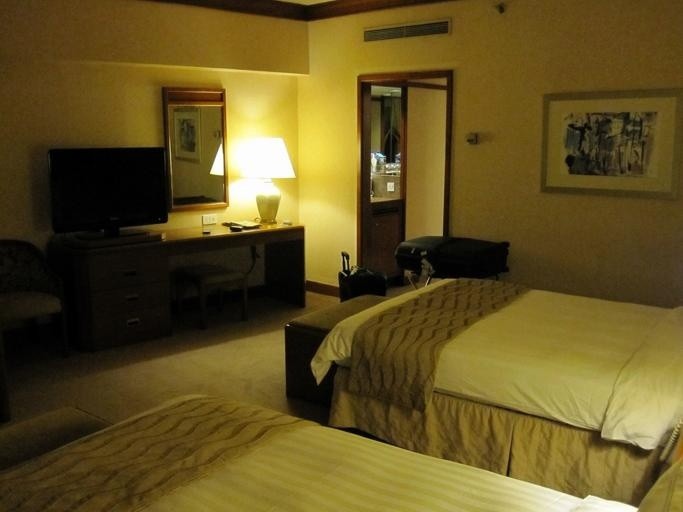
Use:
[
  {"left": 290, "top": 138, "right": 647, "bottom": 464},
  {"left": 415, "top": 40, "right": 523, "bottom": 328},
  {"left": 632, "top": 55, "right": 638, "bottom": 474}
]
[
  {"left": 332, "top": 275, "right": 683, "bottom": 505},
  {"left": 1, "top": 402, "right": 683, "bottom": 512}
]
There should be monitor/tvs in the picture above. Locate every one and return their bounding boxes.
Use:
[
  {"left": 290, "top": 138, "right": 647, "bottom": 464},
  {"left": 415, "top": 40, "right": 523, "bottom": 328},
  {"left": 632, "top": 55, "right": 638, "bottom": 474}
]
[{"left": 48, "top": 146, "right": 171, "bottom": 237}]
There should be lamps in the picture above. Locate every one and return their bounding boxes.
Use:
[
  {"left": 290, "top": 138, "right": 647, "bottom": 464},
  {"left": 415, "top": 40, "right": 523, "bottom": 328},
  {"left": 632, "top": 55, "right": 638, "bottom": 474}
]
[{"left": 238, "top": 136, "right": 297, "bottom": 226}]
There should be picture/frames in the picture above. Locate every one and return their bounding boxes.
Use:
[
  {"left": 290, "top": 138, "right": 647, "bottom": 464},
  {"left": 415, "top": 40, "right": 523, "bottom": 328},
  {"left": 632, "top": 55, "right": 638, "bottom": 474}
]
[
  {"left": 538, "top": 86, "right": 682, "bottom": 202},
  {"left": 172, "top": 107, "right": 201, "bottom": 165}
]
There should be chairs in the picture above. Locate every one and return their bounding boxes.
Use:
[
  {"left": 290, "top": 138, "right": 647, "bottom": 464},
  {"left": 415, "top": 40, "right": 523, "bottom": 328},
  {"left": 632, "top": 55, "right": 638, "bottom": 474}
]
[{"left": 0, "top": 238, "right": 70, "bottom": 374}]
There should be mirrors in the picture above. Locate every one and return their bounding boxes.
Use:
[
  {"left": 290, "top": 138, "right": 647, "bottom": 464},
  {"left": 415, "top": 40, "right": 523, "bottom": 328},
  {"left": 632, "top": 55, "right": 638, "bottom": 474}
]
[
  {"left": 367, "top": 83, "right": 406, "bottom": 204},
  {"left": 161, "top": 87, "right": 230, "bottom": 212}
]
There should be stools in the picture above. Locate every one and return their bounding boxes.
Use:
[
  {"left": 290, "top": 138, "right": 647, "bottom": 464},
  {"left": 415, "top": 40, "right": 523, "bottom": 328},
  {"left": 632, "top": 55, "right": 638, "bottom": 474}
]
[{"left": 172, "top": 266, "right": 248, "bottom": 328}]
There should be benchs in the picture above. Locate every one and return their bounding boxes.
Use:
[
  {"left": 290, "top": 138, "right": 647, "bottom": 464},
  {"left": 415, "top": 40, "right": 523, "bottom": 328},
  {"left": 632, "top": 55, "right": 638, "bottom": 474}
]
[
  {"left": 284, "top": 292, "right": 390, "bottom": 403},
  {"left": 0, "top": 404, "right": 112, "bottom": 469}
]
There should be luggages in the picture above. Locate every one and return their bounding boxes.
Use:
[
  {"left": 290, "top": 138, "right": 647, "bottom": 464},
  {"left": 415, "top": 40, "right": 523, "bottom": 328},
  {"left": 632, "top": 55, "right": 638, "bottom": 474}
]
[
  {"left": 338, "top": 252, "right": 387, "bottom": 301},
  {"left": 395, "top": 236, "right": 510, "bottom": 279}
]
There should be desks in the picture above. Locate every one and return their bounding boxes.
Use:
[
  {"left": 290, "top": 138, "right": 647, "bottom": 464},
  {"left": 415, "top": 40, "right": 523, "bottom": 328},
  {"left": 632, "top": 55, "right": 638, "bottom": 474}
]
[{"left": 46, "top": 218, "right": 306, "bottom": 351}]
